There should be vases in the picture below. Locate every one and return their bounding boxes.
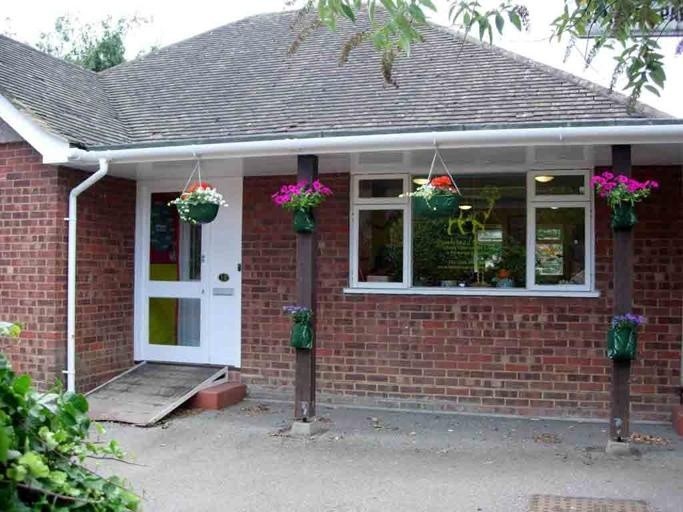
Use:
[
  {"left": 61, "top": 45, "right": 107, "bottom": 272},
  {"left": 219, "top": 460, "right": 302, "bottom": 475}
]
[
  {"left": 188, "top": 204, "right": 220, "bottom": 223},
  {"left": 607, "top": 328, "right": 637, "bottom": 361},
  {"left": 609, "top": 199, "right": 640, "bottom": 229},
  {"left": 412, "top": 194, "right": 465, "bottom": 216},
  {"left": 290, "top": 205, "right": 316, "bottom": 234},
  {"left": 288, "top": 318, "right": 314, "bottom": 351}
]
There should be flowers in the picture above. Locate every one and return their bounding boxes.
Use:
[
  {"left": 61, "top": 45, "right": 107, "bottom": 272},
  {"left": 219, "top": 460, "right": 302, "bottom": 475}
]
[
  {"left": 397, "top": 175, "right": 458, "bottom": 209},
  {"left": 280, "top": 305, "right": 314, "bottom": 323},
  {"left": 167, "top": 179, "right": 229, "bottom": 225},
  {"left": 270, "top": 178, "right": 334, "bottom": 209},
  {"left": 612, "top": 312, "right": 648, "bottom": 328},
  {"left": 590, "top": 170, "right": 660, "bottom": 210}
]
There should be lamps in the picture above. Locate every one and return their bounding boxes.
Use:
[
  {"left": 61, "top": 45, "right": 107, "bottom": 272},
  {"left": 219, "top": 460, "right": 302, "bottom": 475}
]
[
  {"left": 459, "top": 201, "right": 473, "bottom": 210},
  {"left": 536, "top": 176, "right": 556, "bottom": 183},
  {"left": 412, "top": 177, "right": 429, "bottom": 186}
]
